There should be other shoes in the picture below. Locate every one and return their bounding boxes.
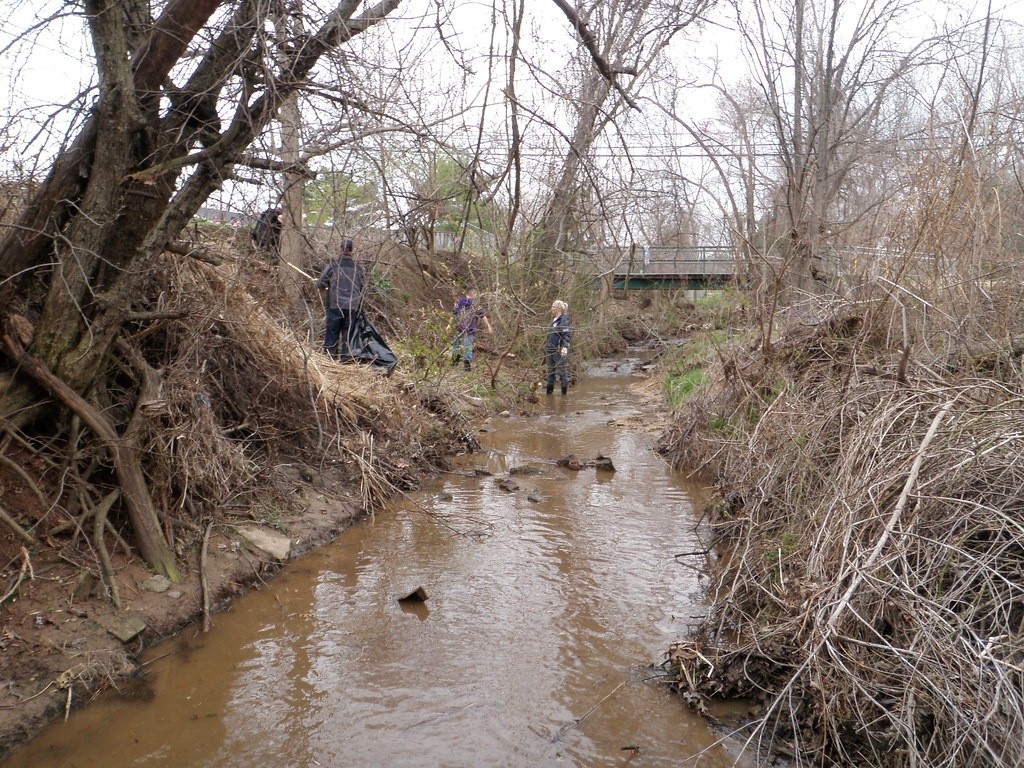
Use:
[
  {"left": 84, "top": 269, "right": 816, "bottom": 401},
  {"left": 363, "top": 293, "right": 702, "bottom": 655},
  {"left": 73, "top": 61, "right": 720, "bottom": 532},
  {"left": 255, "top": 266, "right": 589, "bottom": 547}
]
[
  {"left": 462, "top": 360, "right": 471, "bottom": 372},
  {"left": 451, "top": 354, "right": 461, "bottom": 366}
]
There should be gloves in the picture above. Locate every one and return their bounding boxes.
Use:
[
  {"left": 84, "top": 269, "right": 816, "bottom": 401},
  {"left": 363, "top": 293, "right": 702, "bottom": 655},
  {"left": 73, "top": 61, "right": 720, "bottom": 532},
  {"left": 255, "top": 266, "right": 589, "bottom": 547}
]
[{"left": 561, "top": 347, "right": 568, "bottom": 359}]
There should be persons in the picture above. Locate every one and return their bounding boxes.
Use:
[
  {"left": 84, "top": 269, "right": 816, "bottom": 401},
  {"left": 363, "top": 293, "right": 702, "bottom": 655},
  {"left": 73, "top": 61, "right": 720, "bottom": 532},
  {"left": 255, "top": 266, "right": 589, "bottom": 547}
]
[
  {"left": 446, "top": 285, "right": 494, "bottom": 372},
  {"left": 546, "top": 300, "right": 571, "bottom": 396},
  {"left": 230, "top": 217, "right": 240, "bottom": 226},
  {"left": 317, "top": 240, "right": 364, "bottom": 365},
  {"left": 251, "top": 207, "right": 282, "bottom": 246}
]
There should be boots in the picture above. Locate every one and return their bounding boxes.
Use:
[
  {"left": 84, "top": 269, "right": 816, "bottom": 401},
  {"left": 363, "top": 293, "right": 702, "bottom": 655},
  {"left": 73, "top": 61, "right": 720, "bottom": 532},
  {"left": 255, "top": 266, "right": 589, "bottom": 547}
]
[
  {"left": 562, "top": 386, "right": 568, "bottom": 395},
  {"left": 546, "top": 383, "right": 554, "bottom": 394}
]
